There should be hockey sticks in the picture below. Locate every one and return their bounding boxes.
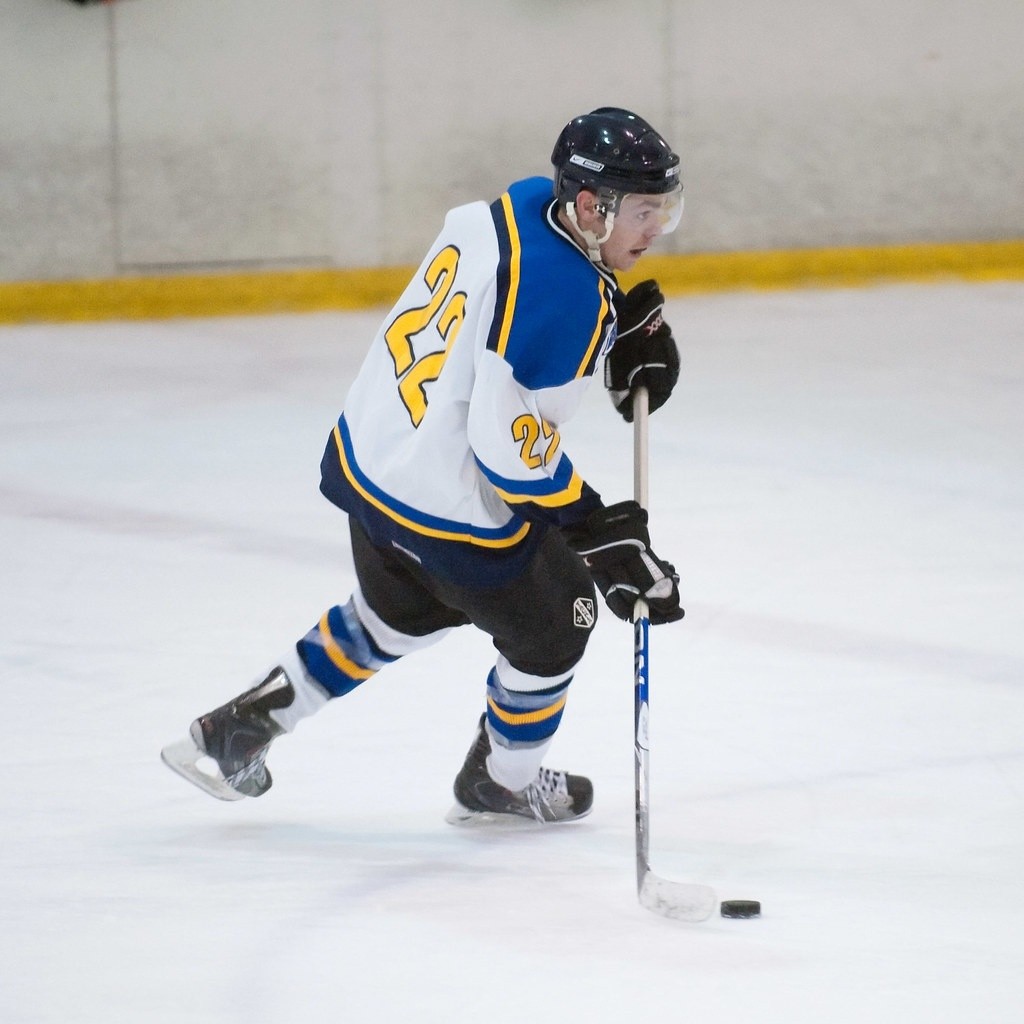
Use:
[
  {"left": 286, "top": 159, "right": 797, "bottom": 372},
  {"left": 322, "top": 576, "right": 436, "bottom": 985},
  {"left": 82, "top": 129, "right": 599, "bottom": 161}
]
[{"left": 613, "top": 372, "right": 722, "bottom": 923}]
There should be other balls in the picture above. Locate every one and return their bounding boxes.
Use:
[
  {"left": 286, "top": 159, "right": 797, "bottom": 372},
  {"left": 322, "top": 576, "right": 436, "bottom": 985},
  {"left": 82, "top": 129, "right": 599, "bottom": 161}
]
[{"left": 720, "top": 900, "right": 761, "bottom": 919}]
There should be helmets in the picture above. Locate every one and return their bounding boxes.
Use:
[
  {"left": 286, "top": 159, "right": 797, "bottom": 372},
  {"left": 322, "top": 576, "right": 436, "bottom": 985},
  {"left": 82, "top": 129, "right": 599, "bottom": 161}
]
[{"left": 549, "top": 107, "right": 681, "bottom": 196}]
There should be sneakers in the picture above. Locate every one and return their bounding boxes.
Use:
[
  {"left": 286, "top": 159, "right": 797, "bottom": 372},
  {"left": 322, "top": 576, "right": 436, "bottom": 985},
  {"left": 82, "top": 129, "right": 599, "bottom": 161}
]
[
  {"left": 160, "top": 662, "right": 296, "bottom": 804},
  {"left": 443, "top": 710, "right": 594, "bottom": 830}
]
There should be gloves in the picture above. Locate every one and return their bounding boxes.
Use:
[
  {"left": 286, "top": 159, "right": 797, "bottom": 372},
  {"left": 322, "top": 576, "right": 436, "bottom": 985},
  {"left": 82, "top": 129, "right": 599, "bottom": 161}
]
[
  {"left": 560, "top": 501, "right": 683, "bottom": 627},
  {"left": 603, "top": 280, "right": 682, "bottom": 423}
]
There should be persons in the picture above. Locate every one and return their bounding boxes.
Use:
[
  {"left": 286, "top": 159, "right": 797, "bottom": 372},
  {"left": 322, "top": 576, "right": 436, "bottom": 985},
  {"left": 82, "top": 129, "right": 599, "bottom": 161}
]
[{"left": 157, "top": 108, "right": 686, "bottom": 824}]
